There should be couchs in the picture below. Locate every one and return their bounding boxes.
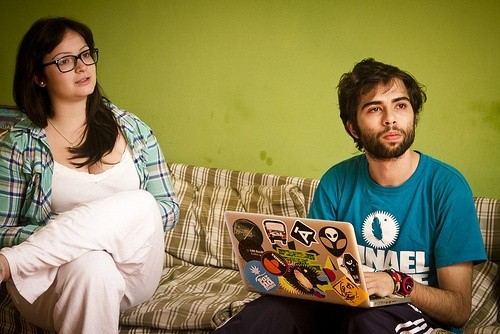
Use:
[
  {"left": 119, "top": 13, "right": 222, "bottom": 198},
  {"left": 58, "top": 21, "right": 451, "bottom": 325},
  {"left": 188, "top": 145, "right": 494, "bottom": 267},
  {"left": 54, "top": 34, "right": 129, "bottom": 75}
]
[{"left": 1, "top": 162, "right": 500, "bottom": 334}]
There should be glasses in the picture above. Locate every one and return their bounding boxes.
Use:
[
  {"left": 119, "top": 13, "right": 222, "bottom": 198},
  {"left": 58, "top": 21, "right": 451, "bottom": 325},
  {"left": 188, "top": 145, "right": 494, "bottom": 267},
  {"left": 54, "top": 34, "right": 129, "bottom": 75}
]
[{"left": 41, "top": 48, "right": 100, "bottom": 74}]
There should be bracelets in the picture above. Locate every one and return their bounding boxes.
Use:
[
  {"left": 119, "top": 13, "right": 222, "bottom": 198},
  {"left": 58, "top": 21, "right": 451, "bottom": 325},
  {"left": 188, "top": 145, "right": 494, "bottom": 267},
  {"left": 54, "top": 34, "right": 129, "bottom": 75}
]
[{"left": 382, "top": 267, "right": 400, "bottom": 296}]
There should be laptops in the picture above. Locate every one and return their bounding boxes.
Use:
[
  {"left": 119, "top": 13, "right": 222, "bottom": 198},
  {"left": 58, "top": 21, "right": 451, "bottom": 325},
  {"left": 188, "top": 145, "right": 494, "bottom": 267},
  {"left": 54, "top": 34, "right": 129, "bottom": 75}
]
[{"left": 224, "top": 211, "right": 412, "bottom": 308}]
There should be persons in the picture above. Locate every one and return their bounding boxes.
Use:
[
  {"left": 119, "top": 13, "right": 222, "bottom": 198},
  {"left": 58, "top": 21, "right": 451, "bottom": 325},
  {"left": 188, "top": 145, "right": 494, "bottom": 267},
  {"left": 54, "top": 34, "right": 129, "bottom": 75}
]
[
  {"left": 216, "top": 58, "right": 489, "bottom": 334},
  {"left": 0, "top": 17, "right": 181, "bottom": 334}
]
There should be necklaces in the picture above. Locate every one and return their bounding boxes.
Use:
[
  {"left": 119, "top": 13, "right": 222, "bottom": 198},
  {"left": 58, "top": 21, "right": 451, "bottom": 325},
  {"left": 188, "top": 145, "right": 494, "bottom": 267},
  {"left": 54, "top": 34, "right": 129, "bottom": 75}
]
[{"left": 47, "top": 117, "right": 81, "bottom": 146}]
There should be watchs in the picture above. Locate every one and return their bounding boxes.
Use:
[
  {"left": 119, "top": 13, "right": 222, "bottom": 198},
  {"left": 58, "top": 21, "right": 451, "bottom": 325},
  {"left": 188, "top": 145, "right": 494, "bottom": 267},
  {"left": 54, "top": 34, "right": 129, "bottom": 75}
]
[{"left": 397, "top": 268, "right": 414, "bottom": 296}]
